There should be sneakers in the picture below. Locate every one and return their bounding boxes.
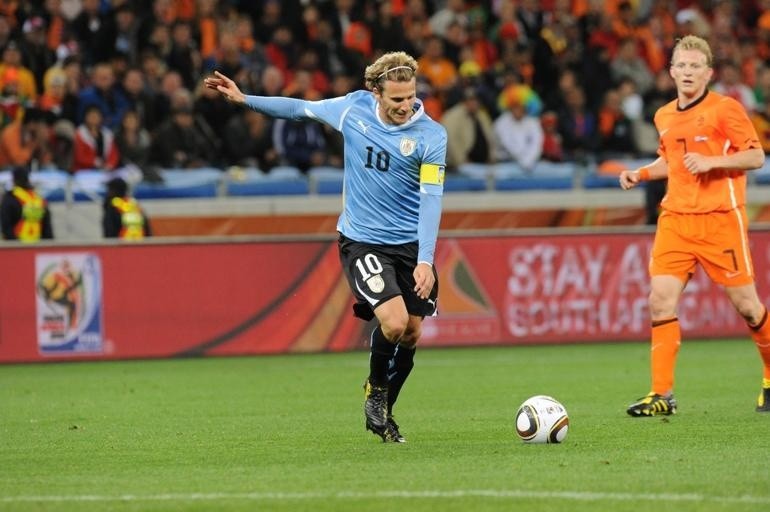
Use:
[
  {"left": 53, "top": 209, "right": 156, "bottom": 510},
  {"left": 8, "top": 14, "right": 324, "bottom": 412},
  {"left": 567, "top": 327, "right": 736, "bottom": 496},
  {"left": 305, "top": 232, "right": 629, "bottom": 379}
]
[
  {"left": 365, "top": 377, "right": 405, "bottom": 443},
  {"left": 628, "top": 392, "right": 678, "bottom": 417},
  {"left": 755, "top": 379, "right": 770, "bottom": 410}
]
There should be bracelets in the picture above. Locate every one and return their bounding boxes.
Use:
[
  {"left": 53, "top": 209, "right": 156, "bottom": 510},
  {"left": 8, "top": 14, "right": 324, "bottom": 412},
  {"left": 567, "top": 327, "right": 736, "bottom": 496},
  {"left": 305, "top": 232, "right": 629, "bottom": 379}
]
[{"left": 638, "top": 168, "right": 651, "bottom": 181}]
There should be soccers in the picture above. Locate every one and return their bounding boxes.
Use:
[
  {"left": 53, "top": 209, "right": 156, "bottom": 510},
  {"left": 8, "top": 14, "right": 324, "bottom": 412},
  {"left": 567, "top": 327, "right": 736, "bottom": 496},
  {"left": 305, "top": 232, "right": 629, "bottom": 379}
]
[{"left": 515, "top": 394, "right": 569, "bottom": 443}]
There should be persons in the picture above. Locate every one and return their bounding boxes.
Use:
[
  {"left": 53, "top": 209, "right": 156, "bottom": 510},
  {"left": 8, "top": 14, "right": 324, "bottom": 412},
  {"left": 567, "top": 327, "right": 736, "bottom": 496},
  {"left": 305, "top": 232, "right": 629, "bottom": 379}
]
[
  {"left": 1, "top": 164, "right": 57, "bottom": 241},
  {"left": 615, "top": 32, "right": 770, "bottom": 420},
  {"left": 201, "top": 47, "right": 446, "bottom": 444},
  {"left": 98, "top": 176, "right": 154, "bottom": 241},
  {"left": 0, "top": 2, "right": 770, "bottom": 176}
]
